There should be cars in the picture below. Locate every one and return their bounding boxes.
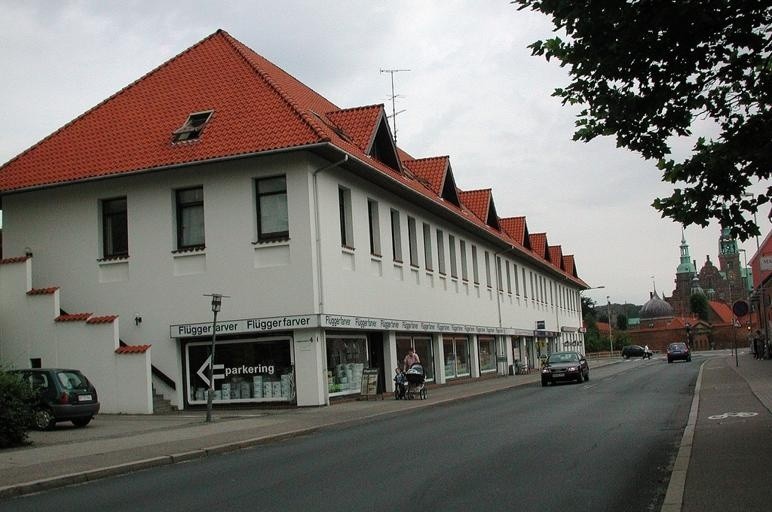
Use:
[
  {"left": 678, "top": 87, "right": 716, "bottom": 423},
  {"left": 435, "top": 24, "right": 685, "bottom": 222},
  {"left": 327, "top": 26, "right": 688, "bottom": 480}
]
[
  {"left": 3, "top": 368, "right": 100, "bottom": 431},
  {"left": 541, "top": 351, "right": 590, "bottom": 387},
  {"left": 667, "top": 341, "right": 691, "bottom": 363},
  {"left": 621, "top": 344, "right": 652, "bottom": 360}
]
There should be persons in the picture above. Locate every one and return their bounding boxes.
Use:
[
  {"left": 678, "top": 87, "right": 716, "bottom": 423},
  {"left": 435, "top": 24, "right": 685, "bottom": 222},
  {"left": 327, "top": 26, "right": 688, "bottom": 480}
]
[
  {"left": 643, "top": 343, "right": 651, "bottom": 359},
  {"left": 403, "top": 348, "right": 420, "bottom": 374},
  {"left": 754, "top": 330, "right": 762, "bottom": 360},
  {"left": 393, "top": 368, "right": 404, "bottom": 396}
]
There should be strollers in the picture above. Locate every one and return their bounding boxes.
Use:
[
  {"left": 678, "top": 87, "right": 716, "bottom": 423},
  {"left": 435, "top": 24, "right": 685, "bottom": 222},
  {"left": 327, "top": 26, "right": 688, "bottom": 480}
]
[{"left": 405, "top": 363, "right": 428, "bottom": 400}]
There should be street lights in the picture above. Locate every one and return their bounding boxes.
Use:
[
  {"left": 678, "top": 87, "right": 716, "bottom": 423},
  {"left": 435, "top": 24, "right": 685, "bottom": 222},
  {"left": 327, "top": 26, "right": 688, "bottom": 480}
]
[
  {"left": 580, "top": 285, "right": 606, "bottom": 292},
  {"left": 729, "top": 280, "right": 739, "bottom": 368},
  {"left": 739, "top": 191, "right": 770, "bottom": 362},
  {"left": 204, "top": 291, "right": 231, "bottom": 424},
  {"left": 606, "top": 296, "right": 614, "bottom": 358}
]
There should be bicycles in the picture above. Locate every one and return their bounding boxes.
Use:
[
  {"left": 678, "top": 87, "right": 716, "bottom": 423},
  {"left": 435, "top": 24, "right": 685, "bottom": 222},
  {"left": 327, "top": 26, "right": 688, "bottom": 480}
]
[{"left": 394, "top": 380, "right": 405, "bottom": 399}]
